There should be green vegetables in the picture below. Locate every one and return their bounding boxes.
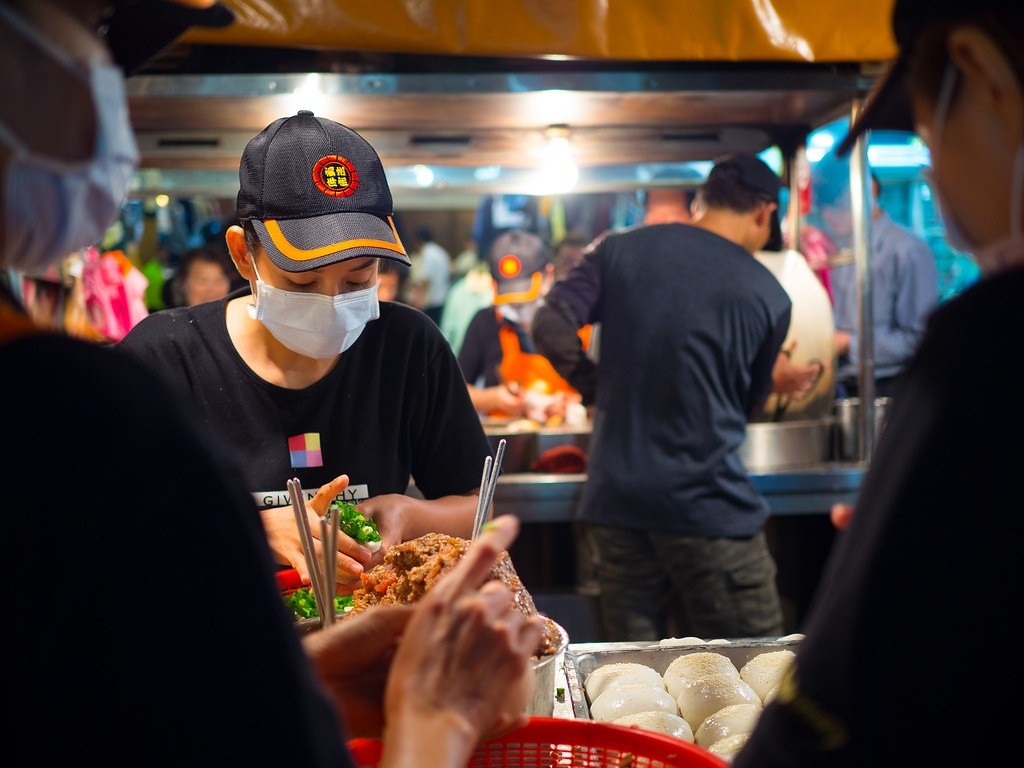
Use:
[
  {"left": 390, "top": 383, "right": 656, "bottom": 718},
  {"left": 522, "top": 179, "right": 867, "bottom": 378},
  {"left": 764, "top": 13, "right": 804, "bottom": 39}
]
[{"left": 285, "top": 501, "right": 382, "bottom": 618}]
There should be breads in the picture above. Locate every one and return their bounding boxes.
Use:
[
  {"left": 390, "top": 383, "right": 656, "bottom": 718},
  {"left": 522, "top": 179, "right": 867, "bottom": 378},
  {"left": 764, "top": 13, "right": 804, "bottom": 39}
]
[{"left": 585, "top": 633, "right": 806, "bottom": 768}]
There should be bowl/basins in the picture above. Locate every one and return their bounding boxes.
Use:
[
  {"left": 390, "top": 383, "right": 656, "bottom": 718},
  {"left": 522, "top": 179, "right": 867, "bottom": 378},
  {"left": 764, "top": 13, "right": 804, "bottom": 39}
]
[
  {"left": 486, "top": 396, "right": 892, "bottom": 473},
  {"left": 298, "top": 613, "right": 571, "bottom": 718}
]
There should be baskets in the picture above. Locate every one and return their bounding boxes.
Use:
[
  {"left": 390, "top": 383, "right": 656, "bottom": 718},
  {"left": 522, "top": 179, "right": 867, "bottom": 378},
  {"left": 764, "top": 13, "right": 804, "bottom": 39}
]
[{"left": 342, "top": 715, "right": 729, "bottom": 768}]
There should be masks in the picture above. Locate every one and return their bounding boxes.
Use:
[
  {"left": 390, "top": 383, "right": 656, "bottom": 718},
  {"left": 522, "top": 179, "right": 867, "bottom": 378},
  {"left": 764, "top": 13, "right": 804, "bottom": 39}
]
[
  {"left": 499, "top": 297, "right": 546, "bottom": 326},
  {"left": 0, "top": 9, "right": 141, "bottom": 275},
  {"left": 925, "top": 67, "right": 1021, "bottom": 264},
  {"left": 249, "top": 251, "right": 383, "bottom": 360}
]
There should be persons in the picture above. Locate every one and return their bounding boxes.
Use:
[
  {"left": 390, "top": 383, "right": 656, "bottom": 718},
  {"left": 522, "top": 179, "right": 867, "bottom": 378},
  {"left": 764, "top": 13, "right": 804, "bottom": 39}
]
[
  {"left": 379, "top": 228, "right": 596, "bottom": 428},
  {"left": 0, "top": 0, "right": 545, "bottom": 768},
  {"left": 115, "top": 110, "right": 504, "bottom": 584},
  {"left": 532, "top": 152, "right": 792, "bottom": 643},
  {"left": 730, "top": 0, "right": 1024, "bottom": 768},
  {"left": 812, "top": 147, "right": 938, "bottom": 398},
  {"left": 182, "top": 249, "right": 231, "bottom": 306},
  {"left": 753, "top": 212, "right": 836, "bottom": 635},
  {"left": 641, "top": 168, "right": 703, "bottom": 225}
]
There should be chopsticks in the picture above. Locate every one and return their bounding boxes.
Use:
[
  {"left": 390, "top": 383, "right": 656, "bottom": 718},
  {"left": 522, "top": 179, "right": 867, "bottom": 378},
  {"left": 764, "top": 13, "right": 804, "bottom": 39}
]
[
  {"left": 471, "top": 436, "right": 507, "bottom": 542},
  {"left": 287, "top": 478, "right": 340, "bottom": 629}
]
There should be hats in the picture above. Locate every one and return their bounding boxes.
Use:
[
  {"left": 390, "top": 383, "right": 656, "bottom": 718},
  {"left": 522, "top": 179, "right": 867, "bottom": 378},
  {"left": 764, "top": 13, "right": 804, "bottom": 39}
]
[
  {"left": 836, "top": 0, "right": 1021, "bottom": 157},
  {"left": 702, "top": 154, "right": 782, "bottom": 251},
  {"left": 102, "top": 0, "right": 234, "bottom": 81},
  {"left": 811, "top": 148, "right": 872, "bottom": 210},
  {"left": 238, "top": 111, "right": 412, "bottom": 272},
  {"left": 489, "top": 228, "right": 549, "bottom": 305}
]
